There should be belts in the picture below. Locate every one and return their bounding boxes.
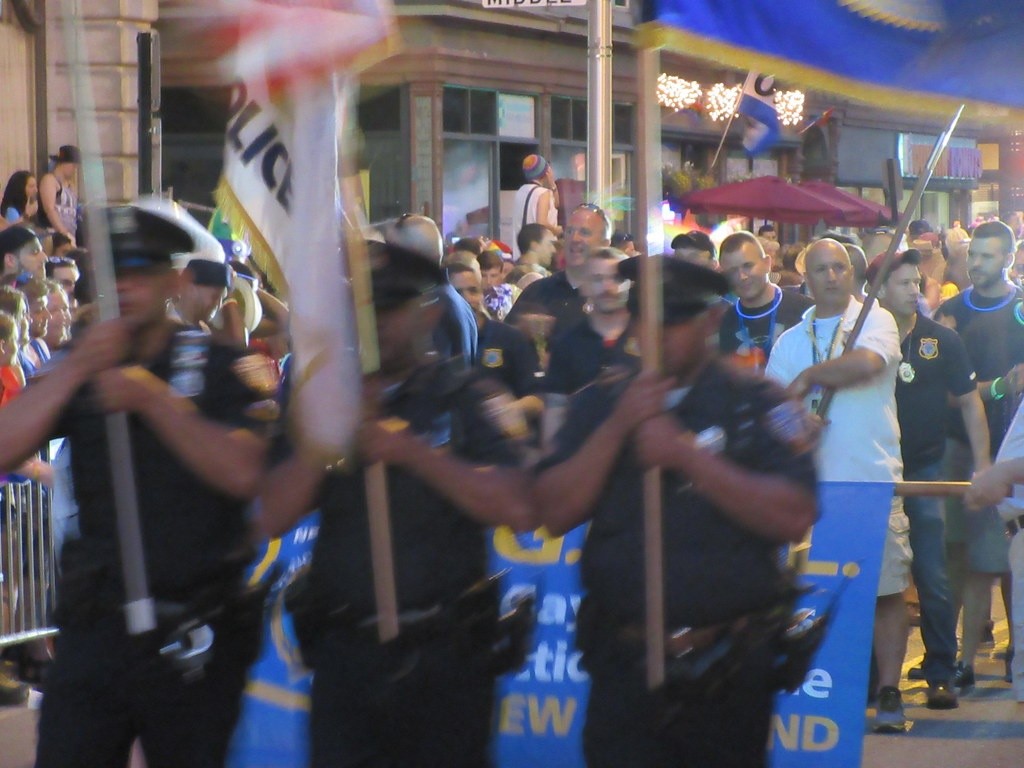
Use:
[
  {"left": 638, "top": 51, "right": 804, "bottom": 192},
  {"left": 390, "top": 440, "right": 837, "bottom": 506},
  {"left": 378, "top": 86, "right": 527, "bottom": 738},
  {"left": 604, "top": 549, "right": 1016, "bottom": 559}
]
[{"left": 1006, "top": 513, "right": 1024, "bottom": 535}]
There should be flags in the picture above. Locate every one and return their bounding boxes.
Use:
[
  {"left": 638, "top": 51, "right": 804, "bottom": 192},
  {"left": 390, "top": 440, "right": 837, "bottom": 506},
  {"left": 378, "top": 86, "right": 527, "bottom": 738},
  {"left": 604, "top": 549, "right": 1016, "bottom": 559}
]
[
  {"left": 734, "top": 69, "right": 779, "bottom": 159},
  {"left": 629, "top": 0, "right": 1024, "bottom": 141}
]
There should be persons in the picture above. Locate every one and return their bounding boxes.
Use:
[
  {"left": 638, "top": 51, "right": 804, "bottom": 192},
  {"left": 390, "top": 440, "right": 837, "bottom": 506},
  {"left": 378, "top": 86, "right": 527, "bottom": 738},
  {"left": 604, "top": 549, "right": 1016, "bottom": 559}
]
[
  {"left": 260, "top": 252, "right": 533, "bottom": 768},
  {"left": 375, "top": 154, "right": 1024, "bottom": 768},
  {"left": 0, "top": 144, "right": 276, "bottom": 502},
  {"left": 0, "top": 200, "right": 281, "bottom": 768},
  {"left": 536, "top": 256, "right": 820, "bottom": 768}
]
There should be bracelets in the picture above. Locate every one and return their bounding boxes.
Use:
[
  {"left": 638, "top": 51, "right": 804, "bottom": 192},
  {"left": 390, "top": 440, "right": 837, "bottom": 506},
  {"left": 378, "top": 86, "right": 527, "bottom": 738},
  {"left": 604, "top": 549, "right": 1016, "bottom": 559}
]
[
  {"left": 991, "top": 377, "right": 1004, "bottom": 400},
  {"left": 550, "top": 186, "right": 558, "bottom": 192}
]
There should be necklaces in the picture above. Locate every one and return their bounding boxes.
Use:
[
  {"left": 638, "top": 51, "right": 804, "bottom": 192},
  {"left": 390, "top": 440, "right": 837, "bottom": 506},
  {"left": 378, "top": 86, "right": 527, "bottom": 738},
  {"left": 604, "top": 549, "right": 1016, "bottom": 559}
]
[
  {"left": 736, "top": 283, "right": 783, "bottom": 320},
  {"left": 964, "top": 281, "right": 1015, "bottom": 311},
  {"left": 581, "top": 296, "right": 595, "bottom": 313},
  {"left": 899, "top": 316, "right": 915, "bottom": 382},
  {"left": 737, "top": 289, "right": 777, "bottom": 360}
]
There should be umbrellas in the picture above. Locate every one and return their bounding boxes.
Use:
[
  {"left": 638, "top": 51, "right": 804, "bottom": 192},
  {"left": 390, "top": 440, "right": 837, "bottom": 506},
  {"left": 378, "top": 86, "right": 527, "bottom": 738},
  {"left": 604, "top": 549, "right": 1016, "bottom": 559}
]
[{"left": 681, "top": 175, "right": 904, "bottom": 229}]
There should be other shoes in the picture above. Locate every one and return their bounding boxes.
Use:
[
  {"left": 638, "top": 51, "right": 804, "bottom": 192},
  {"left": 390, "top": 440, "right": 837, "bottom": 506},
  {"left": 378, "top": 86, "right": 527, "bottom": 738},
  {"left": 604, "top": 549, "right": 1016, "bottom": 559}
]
[
  {"left": 954, "top": 631, "right": 995, "bottom": 649},
  {"left": 874, "top": 685, "right": 904, "bottom": 733},
  {"left": 954, "top": 661, "right": 975, "bottom": 687},
  {"left": 908, "top": 661, "right": 926, "bottom": 680},
  {"left": 925, "top": 677, "right": 956, "bottom": 708}
]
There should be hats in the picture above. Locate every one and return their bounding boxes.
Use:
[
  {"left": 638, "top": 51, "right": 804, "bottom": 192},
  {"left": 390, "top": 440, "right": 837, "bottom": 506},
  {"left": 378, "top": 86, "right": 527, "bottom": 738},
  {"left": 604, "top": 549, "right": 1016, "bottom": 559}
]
[
  {"left": 75, "top": 205, "right": 196, "bottom": 260},
  {"left": 617, "top": 254, "right": 729, "bottom": 321},
  {"left": 523, "top": 154, "right": 548, "bottom": 180},
  {"left": 363, "top": 238, "right": 447, "bottom": 312},
  {"left": 49, "top": 145, "right": 81, "bottom": 163},
  {"left": 230, "top": 276, "right": 263, "bottom": 335}
]
